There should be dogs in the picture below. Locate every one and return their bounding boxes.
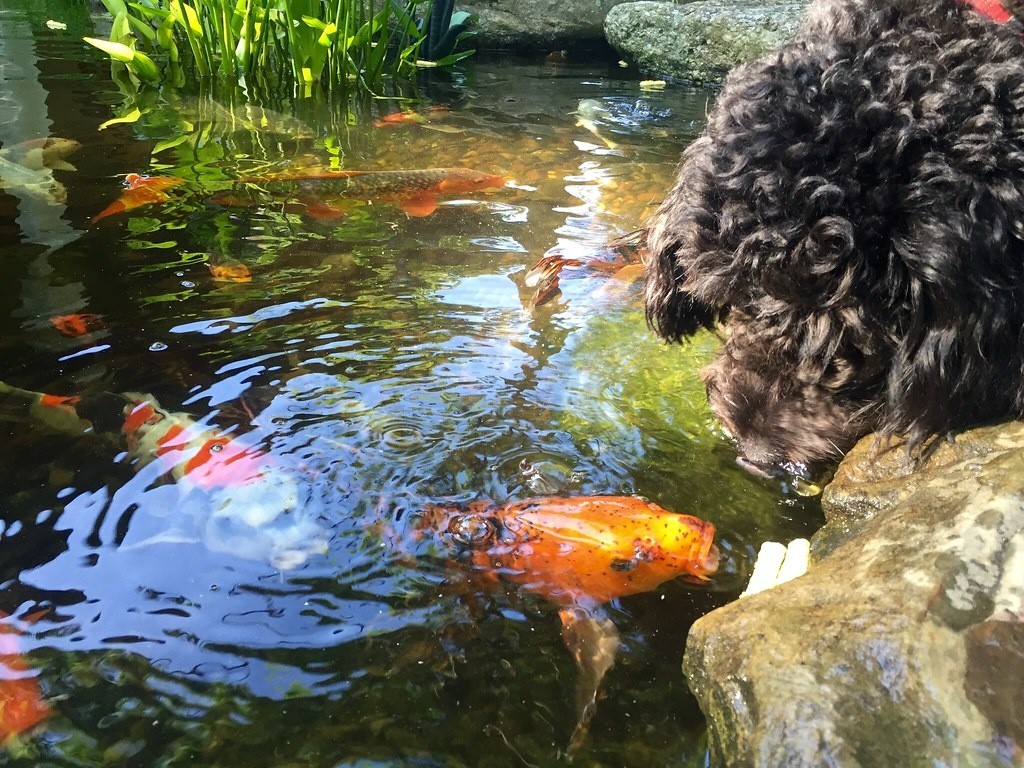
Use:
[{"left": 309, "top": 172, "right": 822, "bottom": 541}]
[{"left": 638, "top": 1, "right": 1024, "bottom": 499}]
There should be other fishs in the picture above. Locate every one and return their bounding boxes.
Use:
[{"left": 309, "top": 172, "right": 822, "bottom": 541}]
[{"left": 0, "top": 97, "right": 728, "bottom": 754}]
